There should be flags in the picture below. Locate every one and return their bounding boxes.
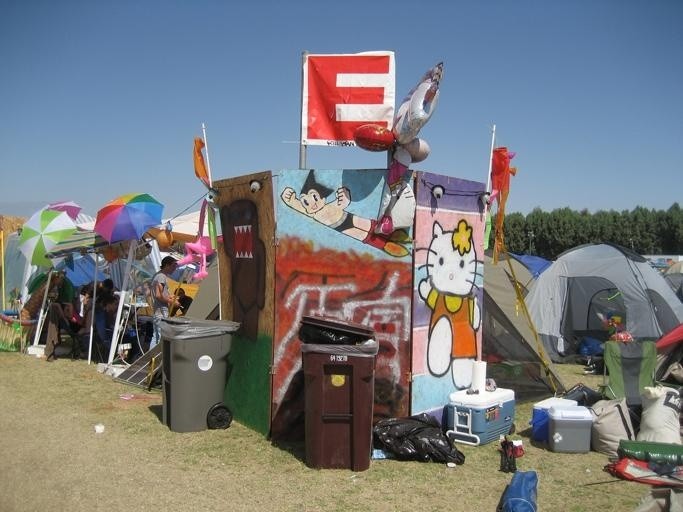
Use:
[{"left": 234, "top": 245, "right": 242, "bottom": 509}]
[{"left": 193, "top": 137, "right": 209, "bottom": 187}]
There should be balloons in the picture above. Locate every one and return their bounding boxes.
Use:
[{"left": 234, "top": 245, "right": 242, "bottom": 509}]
[{"left": 353, "top": 60, "right": 444, "bottom": 163}]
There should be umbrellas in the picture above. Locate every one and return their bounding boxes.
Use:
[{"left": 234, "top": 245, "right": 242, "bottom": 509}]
[
  {"left": 15, "top": 210, "right": 76, "bottom": 269},
  {"left": 43, "top": 200, "right": 83, "bottom": 222},
  {"left": 94, "top": 192, "right": 165, "bottom": 241}
]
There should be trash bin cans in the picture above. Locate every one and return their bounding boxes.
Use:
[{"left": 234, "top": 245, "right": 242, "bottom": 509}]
[
  {"left": 159, "top": 316, "right": 241, "bottom": 433},
  {"left": 298, "top": 313, "right": 379, "bottom": 472}
]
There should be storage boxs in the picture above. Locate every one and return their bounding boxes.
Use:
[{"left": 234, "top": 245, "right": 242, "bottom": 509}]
[
  {"left": 533, "top": 398, "right": 592, "bottom": 453},
  {"left": 447, "top": 387, "right": 516, "bottom": 446}
]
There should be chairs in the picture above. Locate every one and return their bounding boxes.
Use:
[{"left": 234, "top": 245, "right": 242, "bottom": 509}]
[
  {"left": 46, "top": 302, "right": 133, "bottom": 366},
  {"left": 599, "top": 341, "right": 681, "bottom": 431}
]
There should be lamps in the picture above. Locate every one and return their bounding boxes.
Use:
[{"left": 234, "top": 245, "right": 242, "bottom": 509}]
[{"left": 431, "top": 184, "right": 491, "bottom": 206}]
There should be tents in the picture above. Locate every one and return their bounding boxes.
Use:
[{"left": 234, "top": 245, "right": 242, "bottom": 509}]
[{"left": 483, "top": 241, "right": 683, "bottom": 398}]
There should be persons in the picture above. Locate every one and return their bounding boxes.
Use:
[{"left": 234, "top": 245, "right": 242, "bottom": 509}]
[
  {"left": 172, "top": 286, "right": 193, "bottom": 318},
  {"left": 148, "top": 255, "right": 178, "bottom": 350},
  {"left": 61, "top": 279, "right": 144, "bottom": 364}
]
